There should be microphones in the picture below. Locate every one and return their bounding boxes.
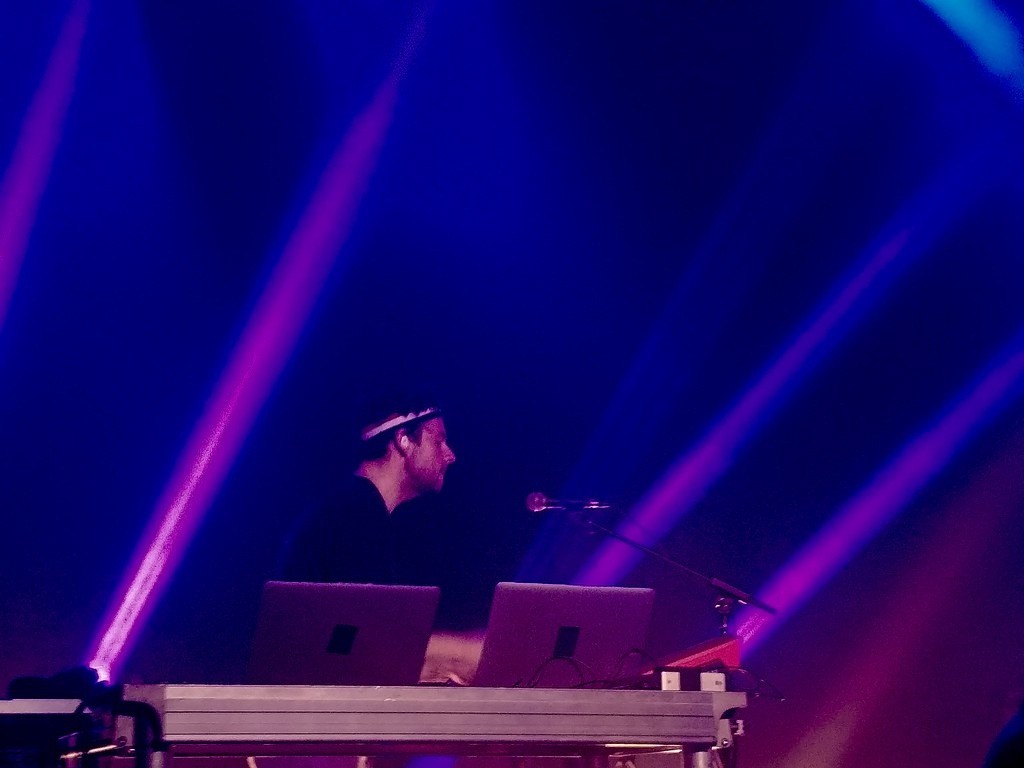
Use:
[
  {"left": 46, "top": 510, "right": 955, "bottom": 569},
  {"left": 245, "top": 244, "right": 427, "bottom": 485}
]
[{"left": 525, "top": 492, "right": 611, "bottom": 512}]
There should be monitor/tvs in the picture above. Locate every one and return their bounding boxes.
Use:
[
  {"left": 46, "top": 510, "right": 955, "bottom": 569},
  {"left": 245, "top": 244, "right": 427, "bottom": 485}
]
[
  {"left": 242, "top": 581, "right": 441, "bottom": 686},
  {"left": 469, "top": 582, "right": 657, "bottom": 691}
]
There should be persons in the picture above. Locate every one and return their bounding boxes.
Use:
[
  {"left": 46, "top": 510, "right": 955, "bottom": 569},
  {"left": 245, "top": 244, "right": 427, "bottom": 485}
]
[{"left": 282, "top": 393, "right": 484, "bottom": 683}]
